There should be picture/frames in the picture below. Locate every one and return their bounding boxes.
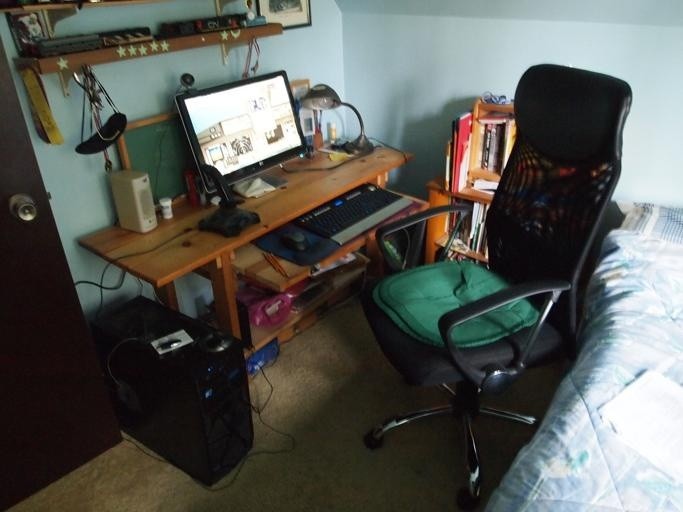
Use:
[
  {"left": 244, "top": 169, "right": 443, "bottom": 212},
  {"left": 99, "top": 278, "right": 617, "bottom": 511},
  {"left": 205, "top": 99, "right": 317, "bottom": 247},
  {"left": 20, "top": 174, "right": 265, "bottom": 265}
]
[{"left": 256, "top": 0, "right": 312, "bottom": 30}]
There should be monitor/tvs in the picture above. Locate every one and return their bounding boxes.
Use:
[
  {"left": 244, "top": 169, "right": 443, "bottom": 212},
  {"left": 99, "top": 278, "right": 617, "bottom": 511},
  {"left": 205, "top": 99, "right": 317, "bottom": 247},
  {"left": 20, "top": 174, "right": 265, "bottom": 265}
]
[{"left": 173, "top": 70, "right": 309, "bottom": 195}]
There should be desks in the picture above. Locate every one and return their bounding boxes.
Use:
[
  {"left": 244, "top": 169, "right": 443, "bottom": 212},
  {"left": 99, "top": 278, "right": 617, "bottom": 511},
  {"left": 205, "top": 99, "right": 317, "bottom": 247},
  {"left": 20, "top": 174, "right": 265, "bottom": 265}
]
[{"left": 76, "top": 137, "right": 430, "bottom": 361}]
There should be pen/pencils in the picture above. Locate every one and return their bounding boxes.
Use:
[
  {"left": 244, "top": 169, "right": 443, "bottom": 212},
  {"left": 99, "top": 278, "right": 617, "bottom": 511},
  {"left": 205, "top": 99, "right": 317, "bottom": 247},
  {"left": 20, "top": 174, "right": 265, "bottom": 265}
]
[{"left": 262, "top": 251, "right": 290, "bottom": 279}]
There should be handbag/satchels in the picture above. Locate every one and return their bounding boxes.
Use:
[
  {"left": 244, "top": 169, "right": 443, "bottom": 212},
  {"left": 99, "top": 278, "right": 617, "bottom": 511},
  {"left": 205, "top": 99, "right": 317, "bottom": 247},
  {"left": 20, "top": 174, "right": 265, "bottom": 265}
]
[{"left": 208, "top": 289, "right": 292, "bottom": 328}]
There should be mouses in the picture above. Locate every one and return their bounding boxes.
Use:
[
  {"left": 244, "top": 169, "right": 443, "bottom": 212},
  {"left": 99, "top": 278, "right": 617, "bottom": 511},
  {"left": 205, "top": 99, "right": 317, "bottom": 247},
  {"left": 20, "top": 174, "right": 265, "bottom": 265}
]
[{"left": 276, "top": 223, "right": 310, "bottom": 252}]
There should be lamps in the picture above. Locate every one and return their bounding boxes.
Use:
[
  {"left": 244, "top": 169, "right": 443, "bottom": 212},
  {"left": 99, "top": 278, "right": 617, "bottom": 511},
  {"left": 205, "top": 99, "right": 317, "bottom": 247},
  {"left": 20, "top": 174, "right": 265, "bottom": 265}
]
[{"left": 299, "top": 83, "right": 374, "bottom": 155}]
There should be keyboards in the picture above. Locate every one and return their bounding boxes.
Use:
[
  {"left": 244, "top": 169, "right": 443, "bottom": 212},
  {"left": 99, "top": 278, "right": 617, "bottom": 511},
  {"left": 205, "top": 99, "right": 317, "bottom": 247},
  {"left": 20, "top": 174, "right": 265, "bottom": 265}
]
[{"left": 296, "top": 180, "right": 413, "bottom": 247}]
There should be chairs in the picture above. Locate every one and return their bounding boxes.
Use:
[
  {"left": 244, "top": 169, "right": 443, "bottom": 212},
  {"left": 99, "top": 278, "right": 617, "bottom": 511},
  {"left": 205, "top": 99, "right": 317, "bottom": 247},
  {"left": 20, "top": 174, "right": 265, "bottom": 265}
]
[{"left": 360, "top": 63, "right": 633, "bottom": 512}]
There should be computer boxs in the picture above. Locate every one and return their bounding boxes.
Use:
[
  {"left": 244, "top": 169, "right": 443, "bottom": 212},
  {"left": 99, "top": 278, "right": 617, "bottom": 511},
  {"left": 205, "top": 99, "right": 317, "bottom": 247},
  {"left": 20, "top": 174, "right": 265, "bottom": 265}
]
[{"left": 87, "top": 292, "right": 256, "bottom": 489}]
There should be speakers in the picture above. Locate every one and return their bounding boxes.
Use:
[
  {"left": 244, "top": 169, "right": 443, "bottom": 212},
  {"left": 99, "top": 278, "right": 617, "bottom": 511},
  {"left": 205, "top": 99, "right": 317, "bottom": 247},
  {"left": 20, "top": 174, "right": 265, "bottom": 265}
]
[{"left": 104, "top": 168, "right": 159, "bottom": 235}]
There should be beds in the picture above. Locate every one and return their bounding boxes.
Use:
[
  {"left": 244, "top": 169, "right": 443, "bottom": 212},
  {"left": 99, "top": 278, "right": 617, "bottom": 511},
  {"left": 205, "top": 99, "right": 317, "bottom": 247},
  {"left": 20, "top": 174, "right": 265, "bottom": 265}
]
[{"left": 497, "top": 201, "right": 683, "bottom": 489}]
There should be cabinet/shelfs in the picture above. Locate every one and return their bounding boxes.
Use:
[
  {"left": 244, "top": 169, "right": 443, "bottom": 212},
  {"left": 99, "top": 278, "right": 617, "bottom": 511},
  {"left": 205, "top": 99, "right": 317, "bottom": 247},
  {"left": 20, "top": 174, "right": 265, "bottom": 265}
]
[
  {"left": 3, "top": 0, "right": 282, "bottom": 98},
  {"left": 426, "top": 96, "right": 516, "bottom": 269}
]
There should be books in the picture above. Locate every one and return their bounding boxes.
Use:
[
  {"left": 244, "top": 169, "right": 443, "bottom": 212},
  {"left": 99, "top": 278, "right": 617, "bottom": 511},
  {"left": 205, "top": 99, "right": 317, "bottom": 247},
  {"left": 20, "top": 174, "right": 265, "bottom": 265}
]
[
  {"left": 440, "top": 196, "right": 490, "bottom": 260},
  {"left": 477, "top": 111, "right": 516, "bottom": 175},
  {"left": 444, "top": 111, "right": 471, "bottom": 194}
]
